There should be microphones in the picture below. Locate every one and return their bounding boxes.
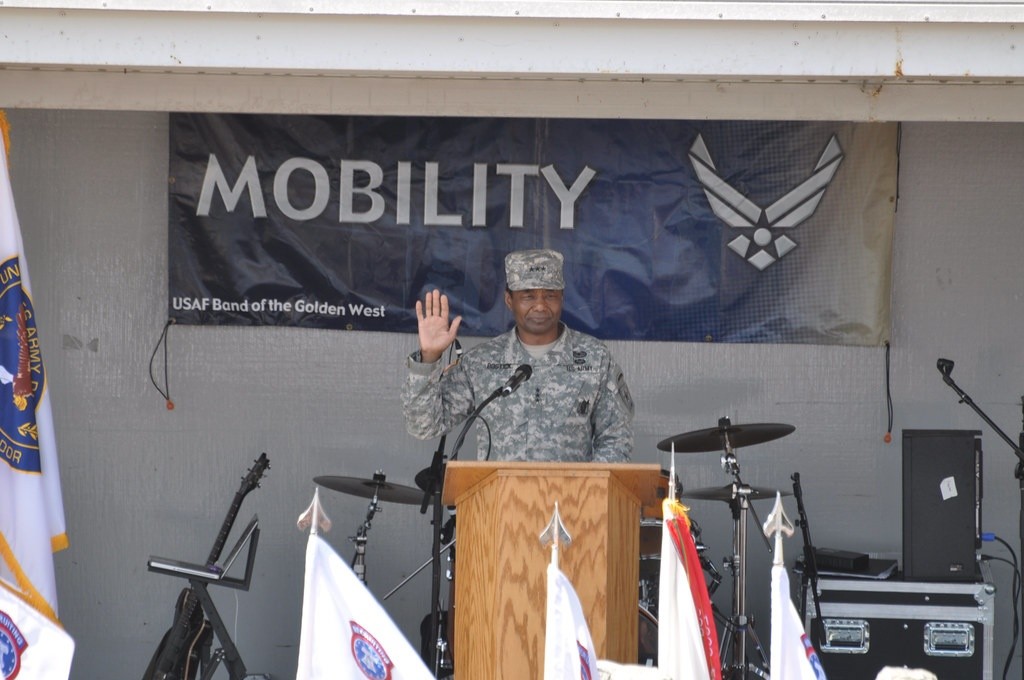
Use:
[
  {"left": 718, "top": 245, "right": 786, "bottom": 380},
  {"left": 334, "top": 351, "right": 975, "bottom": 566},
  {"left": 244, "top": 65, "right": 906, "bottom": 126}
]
[{"left": 502, "top": 364, "right": 533, "bottom": 397}]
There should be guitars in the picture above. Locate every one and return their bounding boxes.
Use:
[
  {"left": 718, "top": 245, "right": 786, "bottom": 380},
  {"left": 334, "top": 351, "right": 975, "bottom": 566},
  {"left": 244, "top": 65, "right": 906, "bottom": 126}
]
[{"left": 140, "top": 452, "right": 271, "bottom": 680}]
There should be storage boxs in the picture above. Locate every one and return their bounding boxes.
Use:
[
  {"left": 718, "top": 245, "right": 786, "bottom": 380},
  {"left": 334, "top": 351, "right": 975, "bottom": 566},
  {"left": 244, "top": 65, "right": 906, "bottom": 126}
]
[{"left": 799, "top": 558, "right": 997, "bottom": 680}]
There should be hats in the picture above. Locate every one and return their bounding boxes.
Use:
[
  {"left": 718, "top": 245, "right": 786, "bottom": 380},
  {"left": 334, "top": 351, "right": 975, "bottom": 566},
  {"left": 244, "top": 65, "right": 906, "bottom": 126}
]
[{"left": 504, "top": 249, "right": 566, "bottom": 291}]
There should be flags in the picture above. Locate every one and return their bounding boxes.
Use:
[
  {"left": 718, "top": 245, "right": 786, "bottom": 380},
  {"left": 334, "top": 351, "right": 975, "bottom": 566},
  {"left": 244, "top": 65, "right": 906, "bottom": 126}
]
[
  {"left": 769, "top": 565, "right": 828, "bottom": 680},
  {"left": 296, "top": 536, "right": 439, "bottom": 680},
  {"left": 542, "top": 564, "right": 599, "bottom": 680},
  {"left": 0, "top": 588, "right": 76, "bottom": 680},
  {"left": 0, "top": 109, "right": 70, "bottom": 619},
  {"left": 657, "top": 497, "right": 721, "bottom": 680}
]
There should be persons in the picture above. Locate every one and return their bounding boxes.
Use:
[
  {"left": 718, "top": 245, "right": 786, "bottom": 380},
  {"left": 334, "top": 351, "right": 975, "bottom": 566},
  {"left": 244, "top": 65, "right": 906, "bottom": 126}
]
[{"left": 399, "top": 249, "right": 634, "bottom": 464}]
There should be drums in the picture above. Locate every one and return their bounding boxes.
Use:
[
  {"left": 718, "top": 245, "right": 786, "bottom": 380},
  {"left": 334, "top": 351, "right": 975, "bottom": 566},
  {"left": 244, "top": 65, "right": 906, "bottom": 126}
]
[
  {"left": 637, "top": 601, "right": 659, "bottom": 668},
  {"left": 638, "top": 469, "right": 679, "bottom": 561},
  {"left": 419, "top": 611, "right": 454, "bottom": 676}
]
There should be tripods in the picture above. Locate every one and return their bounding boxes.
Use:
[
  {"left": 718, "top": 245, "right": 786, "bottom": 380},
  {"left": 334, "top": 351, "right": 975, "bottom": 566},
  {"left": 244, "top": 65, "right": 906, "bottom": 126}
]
[{"left": 718, "top": 430, "right": 769, "bottom": 680}]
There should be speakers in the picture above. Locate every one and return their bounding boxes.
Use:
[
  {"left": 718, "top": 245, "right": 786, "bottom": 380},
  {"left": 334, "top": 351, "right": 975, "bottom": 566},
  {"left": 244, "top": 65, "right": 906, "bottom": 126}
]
[{"left": 901, "top": 428, "right": 984, "bottom": 581}]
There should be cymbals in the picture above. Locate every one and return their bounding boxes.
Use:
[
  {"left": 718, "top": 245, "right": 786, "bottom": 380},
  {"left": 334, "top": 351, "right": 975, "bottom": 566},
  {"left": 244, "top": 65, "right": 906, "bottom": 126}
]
[
  {"left": 678, "top": 483, "right": 793, "bottom": 502},
  {"left": 312, "top": 475, "right": 436, "bottom": 507},
  {"left": 656, "top": 423, "right": 795, "bottom": 453}
]
